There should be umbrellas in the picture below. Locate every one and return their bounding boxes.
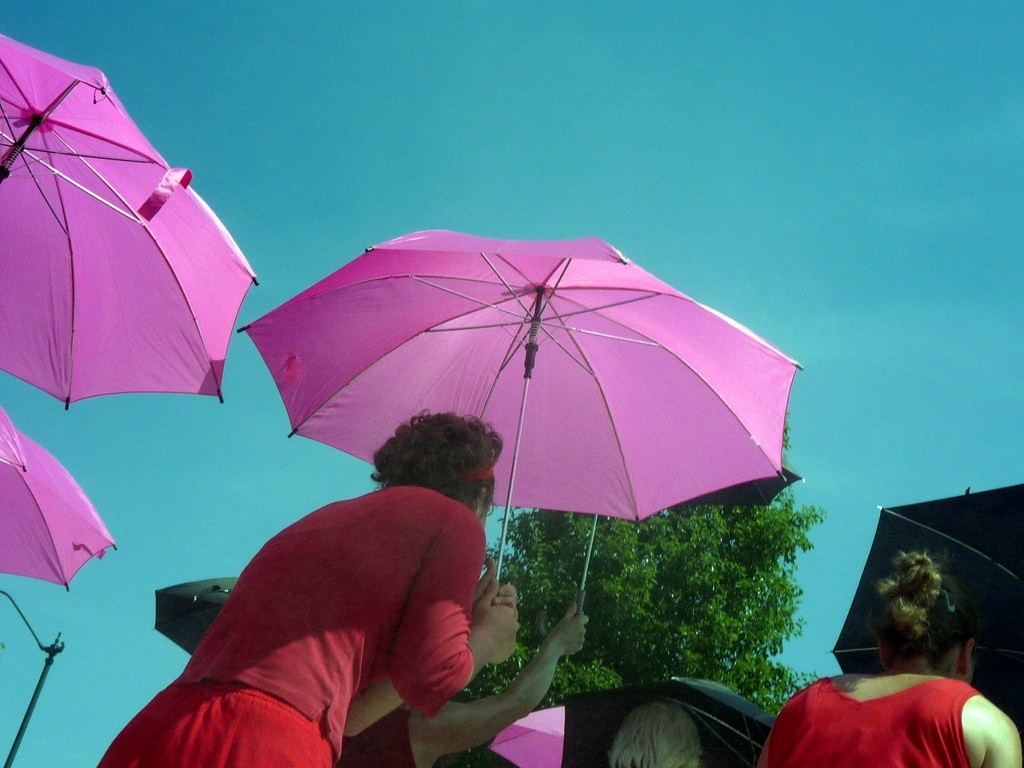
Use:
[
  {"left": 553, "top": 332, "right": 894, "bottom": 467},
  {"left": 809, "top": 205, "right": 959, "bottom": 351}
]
[
  {"left": 489, "top": 705, "right": 565, "bottom": 768},
  {"left": 559, "top": 676, "right": 777, "bottom": 768},
  {"left": 0, "top": 406, "right": 26, "bottom": 472},
  {"left": 830, "top": 483, "right": 1023, "bottom": 768},
  {"left": 234, "top": 230, "right": 804, "bottom": 579},
  {"left": 579, "top": 467, "right": 801, "bottom": 617},
  {"left": 149, "top": 576, "right": 240, "bottom": 657},
  {"left": 0, "top": 34, "right": 259, "bottom": 411},
  {"left": 0, "top": 427, "right": 117, "bottom": 591}
]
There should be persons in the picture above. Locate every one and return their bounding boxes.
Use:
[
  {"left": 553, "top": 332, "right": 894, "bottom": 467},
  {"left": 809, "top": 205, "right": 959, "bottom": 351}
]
[
  {"left": 608, "top": 697, "right": 705, "bottom": 768},
  {"left": 336, "top": 600, "right": 590, "bottom": 768},
  {"left": 756, "top": 550, "right": 1024, "bottom": 768},
  {"left": 96, "top": 409, "right": 521, "bottom": 768}
]
